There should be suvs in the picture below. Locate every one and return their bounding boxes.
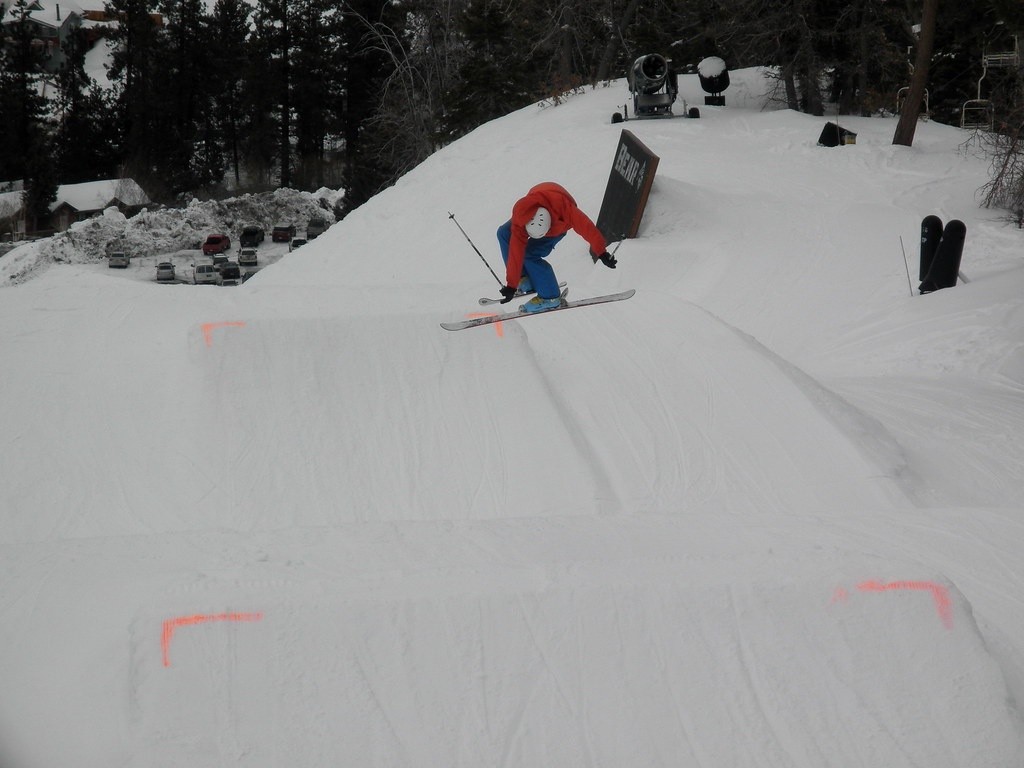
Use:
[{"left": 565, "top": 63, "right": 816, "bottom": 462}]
[{"left": 236, "top": 246, "right": 258, "bottom": 266}]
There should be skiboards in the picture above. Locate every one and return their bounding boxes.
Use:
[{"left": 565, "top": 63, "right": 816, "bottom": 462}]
[{"left": 439, "top": 282, "right": 636, "bottom": 331}]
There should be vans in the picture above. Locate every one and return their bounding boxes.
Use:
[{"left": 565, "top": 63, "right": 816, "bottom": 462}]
[{"left": 191, "top": 259, "right": 216, "bottom": 285}]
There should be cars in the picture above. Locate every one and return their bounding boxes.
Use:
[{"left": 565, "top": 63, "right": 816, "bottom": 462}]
[
  {"left": 218, "top": 279, "right": 238, "bottom": 286},
  {"left": 288, "top": 236, "right": 307, "bottom": 253},
  {"left": 239, "top": 226, "right": 265, "bottom": 248},
  {"left": 240, "top": 271, "right": 257, "bottom": 284},
  {"left": 155, "top": 263, "right": 176, "bottom": 281},
  {"left": 210, "top": 253, "right": 229, "bottom": 272},
  {"left": 307, "top": 217, "right": 330, "bottom": 240},
  {"left": 272, "top": 222, "right": 297, "bottom": 242},
  {"left": 109, "top": 251, "right": 130, "bottom": 269},
  {"left": 219, "top": 261, "right": 240, "bottom": 280}
]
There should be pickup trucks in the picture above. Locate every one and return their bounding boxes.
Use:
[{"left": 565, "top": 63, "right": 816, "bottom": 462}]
[{"left": 203, "top": 233, "right": 230, "bottom": 255}]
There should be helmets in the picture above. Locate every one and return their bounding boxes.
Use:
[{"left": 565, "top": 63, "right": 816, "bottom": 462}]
[{"left": 526, "top": 206, "right": 552, "bottom": 239}]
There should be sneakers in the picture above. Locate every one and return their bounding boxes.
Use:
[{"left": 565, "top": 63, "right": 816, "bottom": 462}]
[
  {"left": 514, "top": 278, "right": 534, "bottom": 298},
  {"left": 524, "top": 296, "right": 561, "bottom": 312}
]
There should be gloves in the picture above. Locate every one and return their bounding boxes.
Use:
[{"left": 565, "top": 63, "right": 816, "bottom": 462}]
[
  {"left": 599, "top": 252, "right": 617, "bottom": 269},
  {"left": 499, "top": 287, "right": 517, "bottom": 304}
]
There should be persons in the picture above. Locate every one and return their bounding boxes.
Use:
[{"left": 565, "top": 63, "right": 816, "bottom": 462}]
[{"left": 496, "top": 183, "right": 617, "bottom": 311}]
